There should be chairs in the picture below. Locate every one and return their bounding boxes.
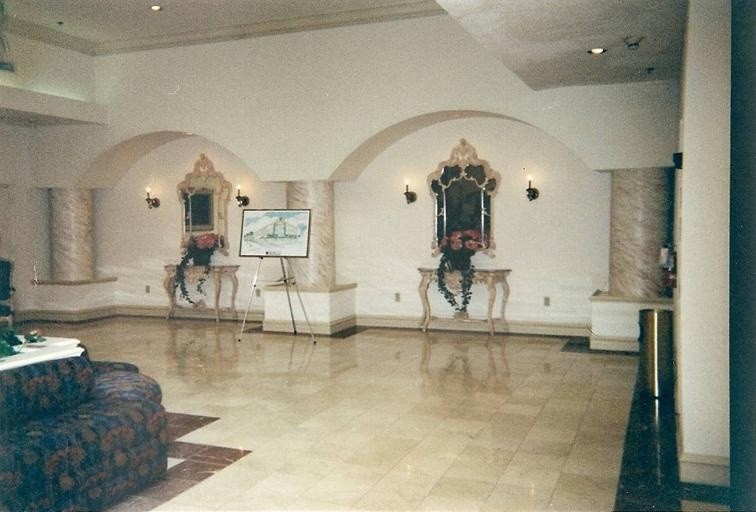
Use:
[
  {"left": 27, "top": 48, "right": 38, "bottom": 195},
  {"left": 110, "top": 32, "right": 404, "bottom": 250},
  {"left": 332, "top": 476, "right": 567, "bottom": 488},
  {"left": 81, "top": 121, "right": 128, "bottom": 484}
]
[{"left": 0, "top": 257, "right": 15, "bottom": 330}]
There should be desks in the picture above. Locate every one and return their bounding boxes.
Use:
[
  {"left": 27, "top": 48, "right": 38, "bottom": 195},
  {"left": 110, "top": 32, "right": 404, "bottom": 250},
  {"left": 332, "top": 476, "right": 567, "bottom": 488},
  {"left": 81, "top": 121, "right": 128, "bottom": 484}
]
[
  {"left": 163, "top": 264, "right": 240, "bottom": 324},
  {"left": 418, "top": 269, "right": 511, "bottom": 336}
]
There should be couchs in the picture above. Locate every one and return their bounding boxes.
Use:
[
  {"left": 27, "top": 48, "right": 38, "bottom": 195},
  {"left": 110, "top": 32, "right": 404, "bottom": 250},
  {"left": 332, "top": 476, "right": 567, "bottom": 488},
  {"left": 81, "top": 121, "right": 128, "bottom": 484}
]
[{"left": 0, "top": 345, "right": 167, "bottom": 512}]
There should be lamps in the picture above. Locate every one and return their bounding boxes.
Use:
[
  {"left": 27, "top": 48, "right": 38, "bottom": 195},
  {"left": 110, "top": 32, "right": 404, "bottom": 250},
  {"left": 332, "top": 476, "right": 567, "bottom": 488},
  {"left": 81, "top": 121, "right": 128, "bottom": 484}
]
[
  {"left": 525, "top": 177, "right": 539, "bottom": 201},
  {"left": 403, "top": 180, "right": 417, "bottom": 204},
  {"left": 145, "top": 187, "right": 160, "bottom": 209},
  {"left": 236, "top": 184, "right": 249, "bottom": 207}
]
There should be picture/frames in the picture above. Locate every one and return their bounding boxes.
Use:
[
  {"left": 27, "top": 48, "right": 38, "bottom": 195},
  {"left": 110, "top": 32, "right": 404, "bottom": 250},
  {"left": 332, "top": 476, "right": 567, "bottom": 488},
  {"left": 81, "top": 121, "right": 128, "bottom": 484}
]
[{"left": 238, "top": 208, "right": 312, "bottom": 258}]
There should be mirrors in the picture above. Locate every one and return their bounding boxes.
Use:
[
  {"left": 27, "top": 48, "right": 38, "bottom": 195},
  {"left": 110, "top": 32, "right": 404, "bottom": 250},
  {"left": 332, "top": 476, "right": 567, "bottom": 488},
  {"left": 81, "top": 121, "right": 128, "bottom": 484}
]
[
  {"left": 177, "top": 154, "right": 232, "bottom": 256},
  {"left": 427, "top": 139, "right": 501, "bottom": 259}
]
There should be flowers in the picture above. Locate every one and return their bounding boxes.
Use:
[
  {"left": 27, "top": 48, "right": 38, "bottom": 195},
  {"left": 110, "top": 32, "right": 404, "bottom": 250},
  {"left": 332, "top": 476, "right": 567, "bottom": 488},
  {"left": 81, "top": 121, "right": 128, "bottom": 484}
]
[
  {"left": 176, "top": 232, "right": 224, "bottom": 307},
  {"left": 432, "top": 230, "right": 489, "bottom": 316}
]
